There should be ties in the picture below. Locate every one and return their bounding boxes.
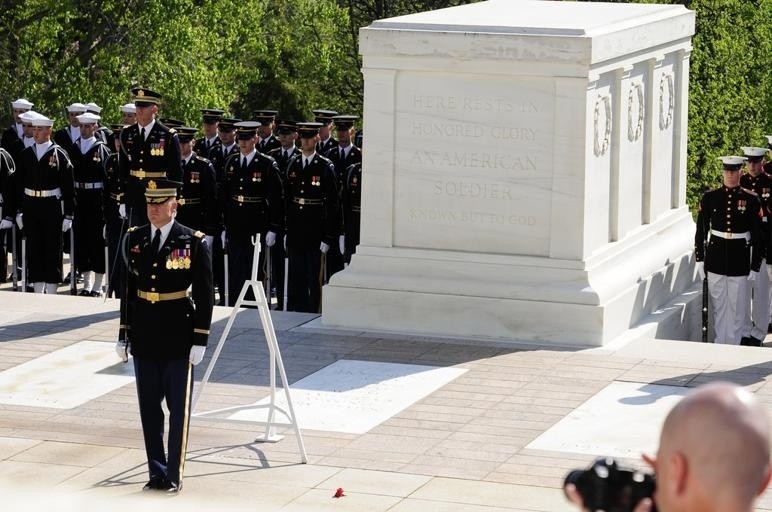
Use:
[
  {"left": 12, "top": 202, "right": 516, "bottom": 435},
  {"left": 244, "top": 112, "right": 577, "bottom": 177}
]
[
  {"left": 223, "top": 147, "right": 229, "bottom": 157},
  {"left": 207, "top": 139, "right": 211, "bottom": 148},
  {"left": 282, "top": 149, "right": 288, "bottom": 162},
  {"left": 152, "top": 227, "right": 162, "bottom": 253},
  {"left": 260, "top": 139, "right": 266, "bottom": 149},
  {"left": 303, "top": 158, "right": 309, "bottom": 169},
  {"left": 181, "top": 158, "right": 188, "bottom": 170},
  {"left": 319, "top": 140, "right": 324, "bottom": 148},
  {"left": 339, "top": 148, "right": 347, "bottom": 161},
  {"left": 241, "top": 155, "right": 248, "bottom": 170}
]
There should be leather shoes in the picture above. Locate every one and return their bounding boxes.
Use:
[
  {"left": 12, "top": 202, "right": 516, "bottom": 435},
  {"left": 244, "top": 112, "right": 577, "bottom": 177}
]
[
  {"left": 143, "top": 479, "right": 165, "bottom": 493},
  {"left": 64, "top": 272, "right": 86, "bottom": 283},
  {"left": 89, "top": 290, "right": 100, "bottom": 297},
  {"left": 160, "top": 480, "right": 184, "bottom": 499},
  {"left": 77, "top": 290, "right": 89, "bottom": 296}
]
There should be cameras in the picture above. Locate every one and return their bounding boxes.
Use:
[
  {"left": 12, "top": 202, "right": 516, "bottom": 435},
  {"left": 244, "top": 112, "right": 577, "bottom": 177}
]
[{"left": 563, "top": 459, "right": 658, "bottom": 512}]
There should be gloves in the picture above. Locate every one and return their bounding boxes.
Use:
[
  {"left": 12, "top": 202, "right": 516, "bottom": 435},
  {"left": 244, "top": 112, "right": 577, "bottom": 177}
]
[
  {"left": 116, "top": 339, "right": 129, "bottom": 363},
  {"left": 117, "top": 202, "right": 128, "bottom": 220},
  {"left": 265, "top": 231, "right": 276, "bottom": 246},
  {"left": 0, "top": 219, "right": 13, "bottom": 229},
  {"left": 319, "top": 241, "right": 329, "bottom": 254},
  {"left": 188, "top": 344, "right": 206, "bottom": 366},
  {"left": 221, "top": 230, "right": 225, "bottom": 249},
  {"left": 339, "top": 235, "right": 345, "bottom": 255},
  {"left": 61, "top": 219, "right": 73, "bottom": 233},
  {"left": 204, "top": 234, "right": 213, "bottom": 246},
  {"left": 15, "top": 212, "right": 24, "bottom": 230}
]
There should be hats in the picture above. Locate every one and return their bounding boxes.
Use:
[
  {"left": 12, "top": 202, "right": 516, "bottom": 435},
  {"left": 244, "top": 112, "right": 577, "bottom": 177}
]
[
  {"left": 159, "top": 109, "right": 360, "bottom": 140},
  {"left": 130, "top": 87, "right": 162, "bottom": 108},
  {"left": 765, "top": 135, "right": 772, "bottom": 150},
  {"left": 716, "top": 156, "right": 749, "bottom": 171},
  {"left": 11, "top": 99, "right": 137, "bottom": 129},
  {"left": 143, "top": 177, "right": 185, "bottom": 204},
  {"left": 740, "top": 146, "right": 770, "bottom": 163}
]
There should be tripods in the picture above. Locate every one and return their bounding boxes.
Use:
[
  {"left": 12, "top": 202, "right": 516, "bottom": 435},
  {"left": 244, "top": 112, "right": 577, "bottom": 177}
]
[{"left": 192, "top": 233, "right": 308, "bottom": 464}]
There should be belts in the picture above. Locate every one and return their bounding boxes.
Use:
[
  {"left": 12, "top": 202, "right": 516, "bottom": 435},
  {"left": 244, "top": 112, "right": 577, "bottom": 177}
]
[
  {"left": 24, "top": 187, "right": 61, "bottom": 198},
  {"left": 351, "top": 205, "right": 362, "bottom": 212},
  {"left": 174, "top": 197, "right": 200, "bottom": 205},
  {"left": 129, "top": 168, "right": 167, "bottom": 180},
  {"left": 73, "top": 180, "right": 104, "bottom": 189},
  {"left": 761, "top": 216, "right": 767, "bottom": 222},
  {"left": 136, "top": 288, "right": 187, "bottom": 302},
  {"left": 230, "top": 193, "right": 263, "bottom": 203},
  {"left": 711, "top": 228, "right": 750, "bottom": 241},
  {"left": 292, "top": 196, "right": 322, "bottom": 206}
]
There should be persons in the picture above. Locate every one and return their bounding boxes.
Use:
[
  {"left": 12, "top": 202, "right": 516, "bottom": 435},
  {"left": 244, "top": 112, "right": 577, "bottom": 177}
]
[
  {"left": 565, "top": 381, "right": 772, "bottom": 511},
  {"left": 115, "top": 177, "right": 217, "bottom": 496},
  {"left": 694, "top": 155, "right": 765, "bottom": 345},
  {"left": 762, "top": 133, "right": 771, "bottom": 175},
  {"left": 737, "top": 145, "right": 772, "bottom": 347},
  {"left": 1, "top": 86, "right": 361, "bottom": 315}
]
